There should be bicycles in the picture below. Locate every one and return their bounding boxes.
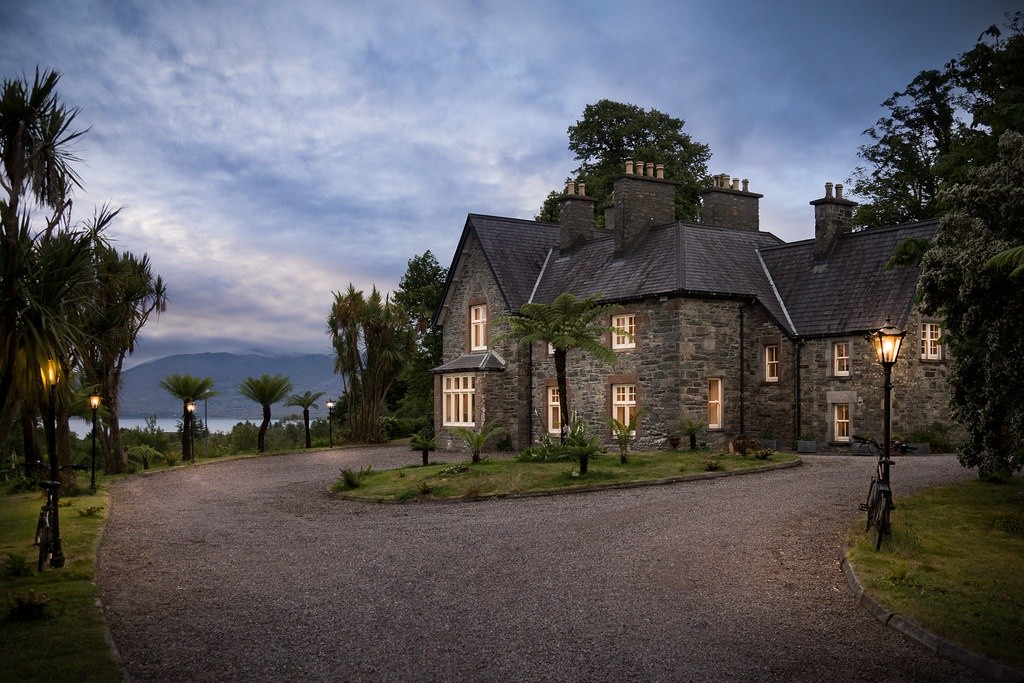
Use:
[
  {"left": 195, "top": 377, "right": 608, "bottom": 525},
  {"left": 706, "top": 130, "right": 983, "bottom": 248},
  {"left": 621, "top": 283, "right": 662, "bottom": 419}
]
[
  {"left": 851, "top": 434, "right": 918, "bottom": 553},
  {"left": 16, "top": 462, "right": 85, "bottom": 573}
]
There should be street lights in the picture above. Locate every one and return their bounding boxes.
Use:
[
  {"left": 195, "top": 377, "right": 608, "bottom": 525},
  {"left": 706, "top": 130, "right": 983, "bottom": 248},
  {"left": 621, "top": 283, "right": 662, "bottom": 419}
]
[
  {"left": 87, "top": 389, "right": 103, "bottom": 489},
  {"left": 38, "top": 358, "right": 64, "bottom": 567},
  {"left": 326, "top": 398, "right": 336, "bottom": 448},
  {"left": 864, "top": 315, "right": 909, "bottom": 509},
  {"left": 186, "top": 401, "right": 197, "bottom": 464}
]
